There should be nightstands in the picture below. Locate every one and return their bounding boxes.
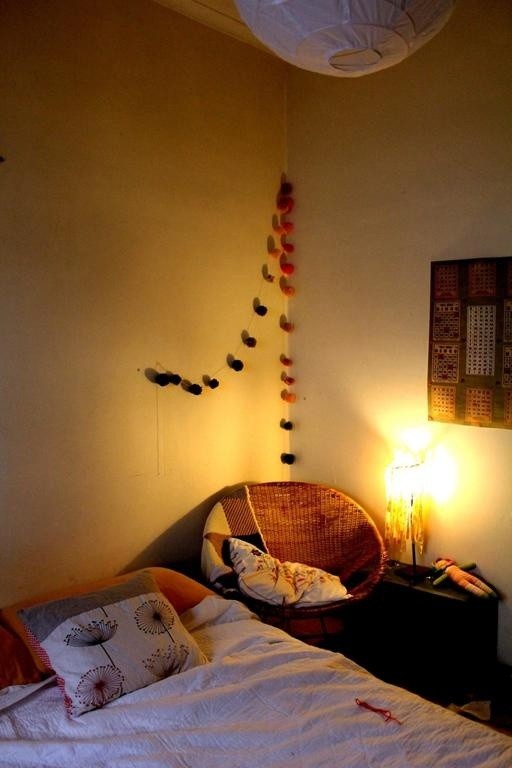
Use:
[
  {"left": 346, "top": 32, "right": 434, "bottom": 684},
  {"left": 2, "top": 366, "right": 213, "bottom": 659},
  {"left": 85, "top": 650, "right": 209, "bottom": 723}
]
[{"left": 374, "top": 557, "right": 499, "bottom": 696}]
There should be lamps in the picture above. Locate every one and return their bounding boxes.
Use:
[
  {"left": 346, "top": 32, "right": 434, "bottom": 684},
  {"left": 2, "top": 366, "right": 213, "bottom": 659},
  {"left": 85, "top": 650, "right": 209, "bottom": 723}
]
[
  {"left": 234, "top": 0, "right": 456, "bottom": 80},
  {"left": 387, "top": 460, "right": 433, "bottom": 584}
]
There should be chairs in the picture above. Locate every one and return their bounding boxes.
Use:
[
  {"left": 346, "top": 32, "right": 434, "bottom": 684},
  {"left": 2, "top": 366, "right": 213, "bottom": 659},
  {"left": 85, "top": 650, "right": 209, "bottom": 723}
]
[{"left": 201, "top": 480, "right": 386, "bottom": 636}]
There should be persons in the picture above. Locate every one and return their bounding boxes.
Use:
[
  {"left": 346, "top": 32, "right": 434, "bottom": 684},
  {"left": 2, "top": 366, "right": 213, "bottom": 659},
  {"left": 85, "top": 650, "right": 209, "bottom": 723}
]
[{"left": 431, "top": 557, "right": 498, "bottom": 600}]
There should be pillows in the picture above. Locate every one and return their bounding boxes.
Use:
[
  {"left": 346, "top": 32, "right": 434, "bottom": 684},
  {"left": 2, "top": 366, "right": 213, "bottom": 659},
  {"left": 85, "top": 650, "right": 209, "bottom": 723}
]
[
  {"left": 0, "top": 567, "right": 230, "bottom": 671},
  {"left": 226, "top": 536, "right": 348, "bottom": 604},
  {"left": 19, "top": 566, "right": 211, "bottom": 721}
]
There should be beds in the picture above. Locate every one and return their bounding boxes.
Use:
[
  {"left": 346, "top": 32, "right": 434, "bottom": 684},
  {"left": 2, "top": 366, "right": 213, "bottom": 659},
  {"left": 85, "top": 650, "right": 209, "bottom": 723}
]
[{"left": 0, "top": 584, "right": 512, "bottom": 768}]
